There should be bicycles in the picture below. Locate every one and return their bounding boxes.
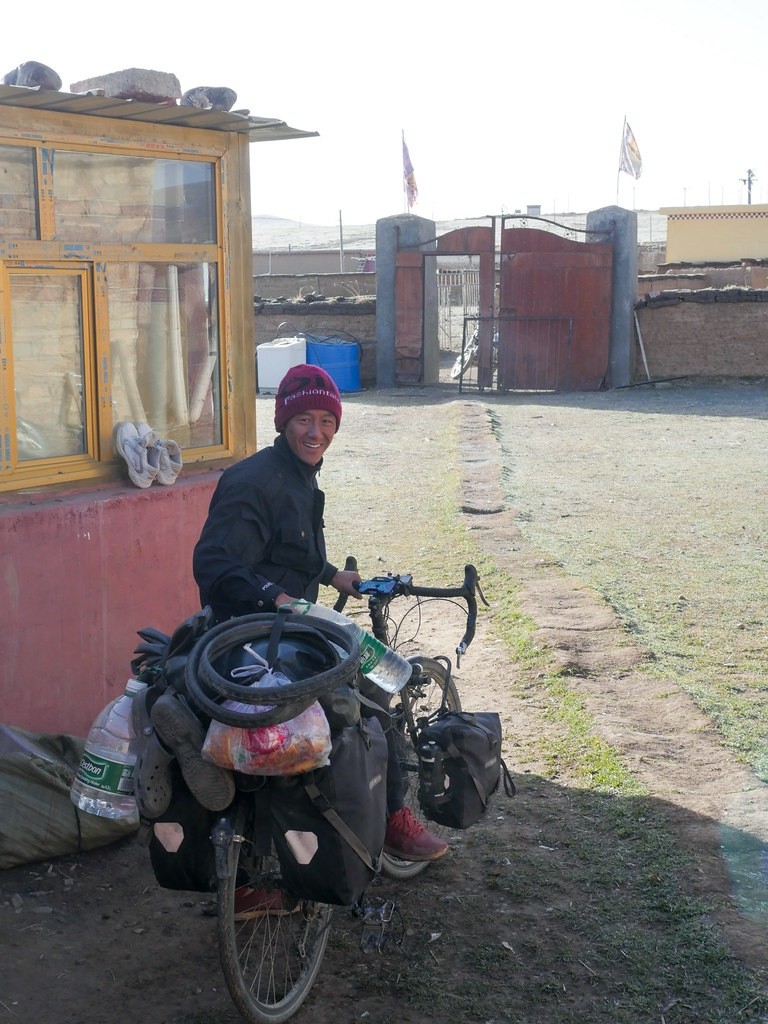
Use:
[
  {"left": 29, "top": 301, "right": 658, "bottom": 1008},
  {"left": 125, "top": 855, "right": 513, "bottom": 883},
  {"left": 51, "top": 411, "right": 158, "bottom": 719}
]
[{"left": 200, "top": 551, "right": 489, "bottom": 1024}]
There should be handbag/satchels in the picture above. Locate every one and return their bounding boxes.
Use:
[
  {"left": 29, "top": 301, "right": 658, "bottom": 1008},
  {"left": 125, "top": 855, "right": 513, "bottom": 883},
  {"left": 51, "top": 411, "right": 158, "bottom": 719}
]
[
  {"left": 200, "top": 643, "right": 332, "bottom": 777},
  {"left": 269, "top": 718, "right": 389, "bottom": 905},
  {"left": 150, "top": 767, "right": 257, "bottom": 891},
  {"left": 416, "top": 711, "right": 516, "bottom": 829}
]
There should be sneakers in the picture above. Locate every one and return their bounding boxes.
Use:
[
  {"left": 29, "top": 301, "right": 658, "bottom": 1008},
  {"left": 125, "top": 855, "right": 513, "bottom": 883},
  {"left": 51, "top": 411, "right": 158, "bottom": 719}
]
[
  {"left": 234, "top": 884, "right": 304, "bottom": 922},
  {"left": 137, "top": 423, "right": 183, "bottom": 486},
  {"left": 382, "top": 808, "right": 448, "bottom": 862},
  {"left": 116, "top": 421, "right": 161, "bottom": 488}
]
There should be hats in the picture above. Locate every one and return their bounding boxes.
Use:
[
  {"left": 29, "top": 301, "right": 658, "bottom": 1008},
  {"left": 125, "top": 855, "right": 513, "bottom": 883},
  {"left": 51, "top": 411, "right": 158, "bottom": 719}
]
[{"left": 274, "top": 366, "right": 341, "bottom": 434}]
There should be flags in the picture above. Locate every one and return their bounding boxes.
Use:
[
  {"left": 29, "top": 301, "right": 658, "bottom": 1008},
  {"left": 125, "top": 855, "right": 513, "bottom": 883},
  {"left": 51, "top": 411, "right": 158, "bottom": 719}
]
[
  {"left": 402, "top": 141, "right": 418, "bottom": 208},
  {"left": 620, "top": 123, "right": 642, "bottom": 178}
]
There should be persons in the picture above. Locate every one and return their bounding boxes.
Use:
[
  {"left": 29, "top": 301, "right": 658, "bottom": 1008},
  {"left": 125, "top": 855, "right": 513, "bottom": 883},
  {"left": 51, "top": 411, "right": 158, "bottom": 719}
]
[{"left": 190, "top": 365, "right": 450, "bottom": 863}]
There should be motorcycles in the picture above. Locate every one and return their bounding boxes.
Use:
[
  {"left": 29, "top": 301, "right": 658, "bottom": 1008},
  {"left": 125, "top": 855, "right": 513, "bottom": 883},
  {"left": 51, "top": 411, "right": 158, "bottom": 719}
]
[{"left": 452, "top": 313, "right": 499, "bottom": 380}]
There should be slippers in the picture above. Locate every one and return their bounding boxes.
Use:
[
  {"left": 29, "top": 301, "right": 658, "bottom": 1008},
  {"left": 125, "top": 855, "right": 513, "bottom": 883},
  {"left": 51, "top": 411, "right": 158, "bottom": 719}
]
[
  {"left": 151, "top": 693, "right": 236, "bottom": 811},
  {"left": 132, "top": 685, "right": 177, "bottom": 818}
]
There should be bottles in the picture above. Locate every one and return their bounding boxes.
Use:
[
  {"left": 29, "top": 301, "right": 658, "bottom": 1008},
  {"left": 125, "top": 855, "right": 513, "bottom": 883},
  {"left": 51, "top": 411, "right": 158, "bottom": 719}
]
[
  {"left": 278, "top": 598, "right": 413, "bottom": 694},
  {"left": 421, "top": 742, "right": 444, "bottom": 797},
  {"left": 69, "top": 679, "right": 149, "bottom": 820}
]
